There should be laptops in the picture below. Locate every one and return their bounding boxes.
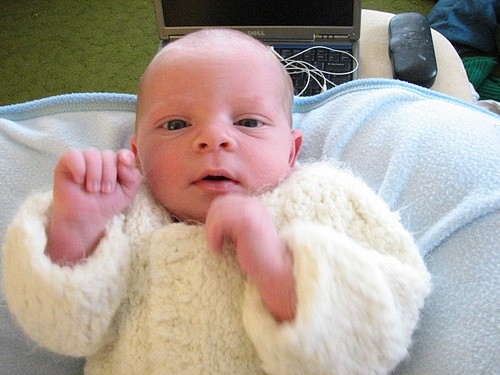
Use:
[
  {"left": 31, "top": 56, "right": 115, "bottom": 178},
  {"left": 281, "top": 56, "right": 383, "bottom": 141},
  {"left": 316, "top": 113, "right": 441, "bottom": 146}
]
[{"left": 153, "top": 1, "right": 360, "bottom": 95}]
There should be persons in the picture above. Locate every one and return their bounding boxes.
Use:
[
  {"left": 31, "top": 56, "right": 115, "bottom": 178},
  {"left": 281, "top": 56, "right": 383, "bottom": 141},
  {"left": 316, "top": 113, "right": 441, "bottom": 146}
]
[{"left": 3, "top": 28, "right": 430, "bottom": 374}]
